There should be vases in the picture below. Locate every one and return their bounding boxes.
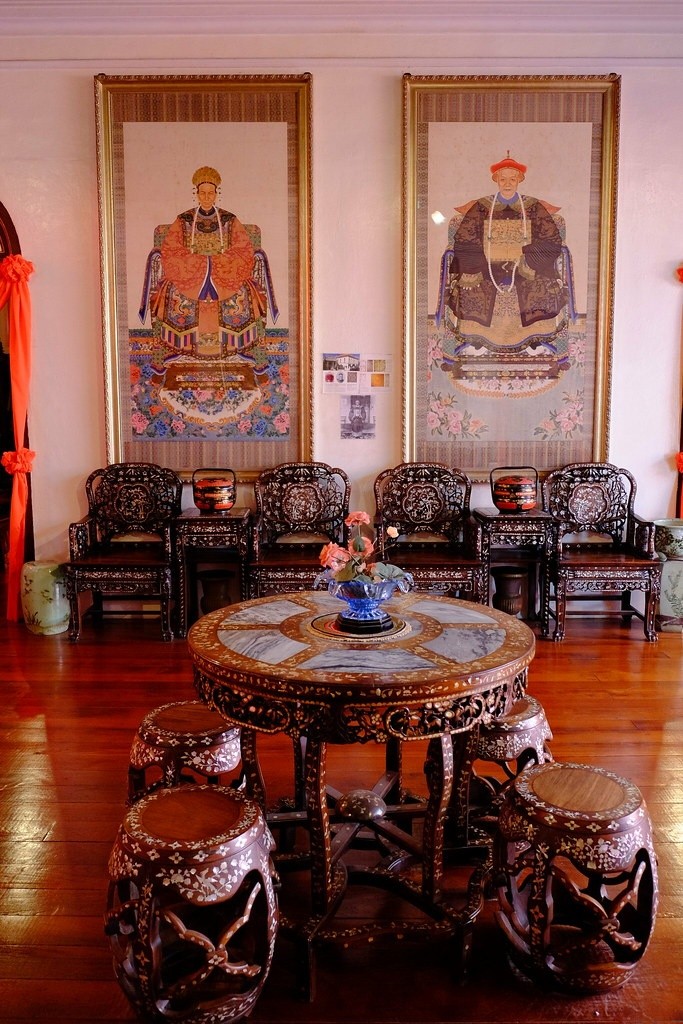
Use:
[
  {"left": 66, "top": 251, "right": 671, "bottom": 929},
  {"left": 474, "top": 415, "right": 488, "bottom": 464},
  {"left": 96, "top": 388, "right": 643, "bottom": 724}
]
[
  {"left": 653, "top": 518, "right": 683, "bottom": 561},
  {"left": 326, "top": 578, "right": 398, "bottom": 635}
]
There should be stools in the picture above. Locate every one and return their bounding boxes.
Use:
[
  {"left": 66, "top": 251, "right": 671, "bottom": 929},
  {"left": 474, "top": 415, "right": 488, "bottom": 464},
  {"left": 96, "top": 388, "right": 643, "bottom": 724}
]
[
  {"left": 127, "top": 700, "right": 249, "bottom": 811},
  {"left": 102, "top": 783, "right": 280, "bottom": 1024},
  {"left": 491, "top": 762, "right": 660, "bottom": 996},
  {"left": 423, "top": 693, "right": 557, "bottom": 866}
]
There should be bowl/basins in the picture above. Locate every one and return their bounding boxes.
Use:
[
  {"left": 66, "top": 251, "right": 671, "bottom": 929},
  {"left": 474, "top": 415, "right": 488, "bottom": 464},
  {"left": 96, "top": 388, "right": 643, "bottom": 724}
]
[{"left": 652, "top": 518, "right": 683, "bottom": 560}]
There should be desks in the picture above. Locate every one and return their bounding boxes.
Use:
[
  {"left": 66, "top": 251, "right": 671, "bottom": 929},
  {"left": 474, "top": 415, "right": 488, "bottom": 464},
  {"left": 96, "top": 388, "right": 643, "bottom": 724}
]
[
  {"left": 172, "top": 507, "right": 251, "bottom": 638},
  {"left": 471, "top": 506, "right": 553, "bottom": 638},
  {"left": 186, "top": 590, "right": 537, "bottom": 1004}
]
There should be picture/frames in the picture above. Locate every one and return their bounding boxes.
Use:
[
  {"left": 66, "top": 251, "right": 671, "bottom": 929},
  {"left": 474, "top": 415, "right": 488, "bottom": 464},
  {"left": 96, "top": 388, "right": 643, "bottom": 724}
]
[
  {"left": 400, "top": 71, "right": 622, "bottom": 485},
  {"left": 93, "top": 72, "right": 314, "bottom": 486}
]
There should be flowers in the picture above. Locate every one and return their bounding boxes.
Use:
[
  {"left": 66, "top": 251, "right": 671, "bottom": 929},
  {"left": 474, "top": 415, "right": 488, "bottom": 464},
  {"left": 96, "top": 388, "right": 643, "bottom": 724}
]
[{"left": 312, "top": 510, "right": 415, "bottom": 594}]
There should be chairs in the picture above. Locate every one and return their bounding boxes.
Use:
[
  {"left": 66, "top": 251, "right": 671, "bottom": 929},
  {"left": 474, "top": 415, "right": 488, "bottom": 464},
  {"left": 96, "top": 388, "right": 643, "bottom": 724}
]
[
  {"left": 538, "top": 462, "right": 664, "bottom": 643},
  {"left": 372, "top": 462, "right": 485, "bottom": 606},
  {"left": 247, "top": 462, "right": 353, "bottom": 599},
  {"left": 57, "top": 462, "right": 183, "bottom": 644}
]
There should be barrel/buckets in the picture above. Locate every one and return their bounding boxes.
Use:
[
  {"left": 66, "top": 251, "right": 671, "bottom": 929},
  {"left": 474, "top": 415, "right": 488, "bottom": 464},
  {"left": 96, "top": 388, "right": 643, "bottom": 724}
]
[
  {"left": 189, "top": 467, "right": 237, "bottom": 514},
  {"left": 490, "top": 466, "right": 538, "bottom": 514}
]
